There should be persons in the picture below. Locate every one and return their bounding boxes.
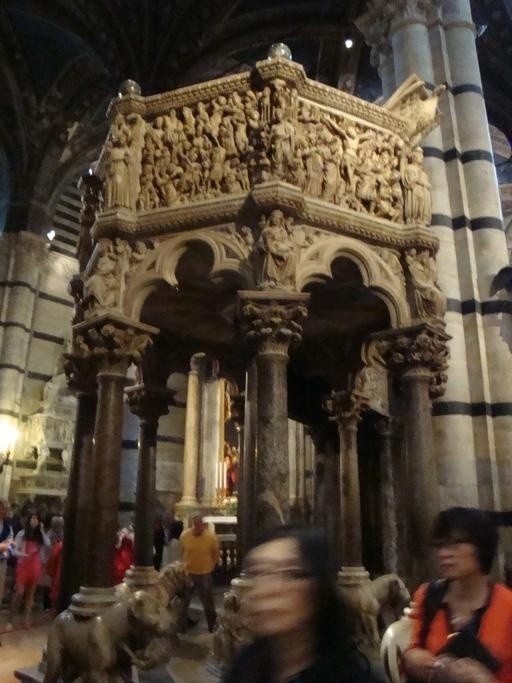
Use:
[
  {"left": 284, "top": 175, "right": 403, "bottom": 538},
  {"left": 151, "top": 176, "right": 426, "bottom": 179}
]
[
  {"left": 79, "top": 117, "right": 133, "bottom": 228},
  {"left": 213, "top": 523, "right": 391, "bottom": 681},
  {"left": 175, "top": 509, "right": 223, "bottom": 636},
  {"left": 82, "top": 234, "right": 128, "bottom": 318},
  {"left": 1, "top": 494, "right": 138, "bottom": 634},
  {"left": 257, "top": 207, "right": 301, "bottom": 291},
  {"left": 138, "top": 83, "right": 432, "bottom": 226},
  {"left": 404, "top": 247, "right": 449, "bottom": 324},
  {"left": 150, "top": 506, "right": 184, "bottom": 571},
  {"left": 393, "top": 503, "right": 512, "bottom": 682}
]
